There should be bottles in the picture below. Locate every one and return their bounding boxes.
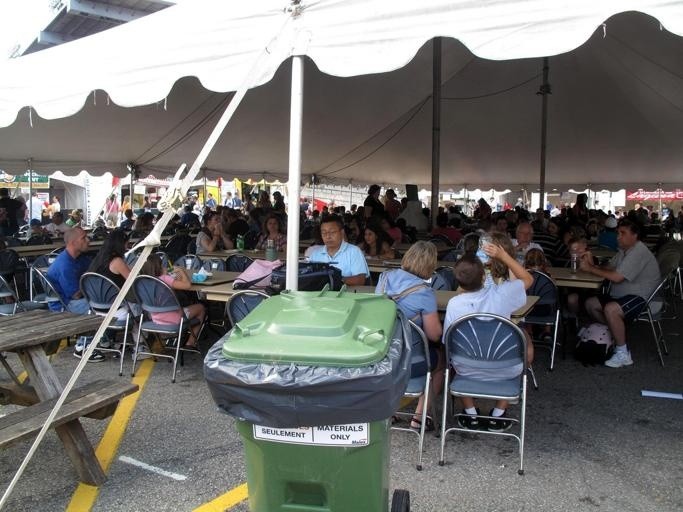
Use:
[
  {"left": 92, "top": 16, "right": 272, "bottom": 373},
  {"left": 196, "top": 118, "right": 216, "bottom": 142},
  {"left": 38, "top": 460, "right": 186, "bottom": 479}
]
[
  {"left": 68, "top": 209, "right": 80, "bottom": 217},
  {"left": 30, "top": 219, "right": 42, "bottom": 227}
]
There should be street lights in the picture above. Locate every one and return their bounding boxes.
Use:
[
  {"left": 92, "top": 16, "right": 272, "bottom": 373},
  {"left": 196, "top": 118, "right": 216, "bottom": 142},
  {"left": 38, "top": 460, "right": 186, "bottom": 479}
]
[{"left": 233, "top": 261, "right": 343, "bottom": 291}]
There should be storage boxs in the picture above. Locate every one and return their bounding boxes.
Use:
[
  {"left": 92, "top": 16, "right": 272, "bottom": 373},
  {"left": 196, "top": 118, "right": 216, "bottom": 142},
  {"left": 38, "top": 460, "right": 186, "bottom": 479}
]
[{"left": 1, "top": 377, "right": 139, "bottom": 453}]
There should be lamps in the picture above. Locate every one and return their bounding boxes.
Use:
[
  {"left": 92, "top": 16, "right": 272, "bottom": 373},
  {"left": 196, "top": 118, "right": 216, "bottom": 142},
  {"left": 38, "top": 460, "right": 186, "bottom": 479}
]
[
  {"left": 156, "top": 252, "right": 169, "bottom": 268},
  {"left": 388, "top": 319, "right": 433, "bottom": 472},
  {"left": 173, "top": 254, "right": 201, "bottom": 272},
  {"left": 436, "top": 265, "right": 459, "bottom": 291},
  {"left": 437, "top": 312, "right": 538, "bottom": 476},
  {"left": 34, "top": 266, "right": 72, "bottom": 312},
  {"left": 633, "top": 267, "right": 680, "bottom": 368},
  {"left": 522, "top": 269, "right": 563, "bottom": 372},
  {"left": 225, "top": 288, "right": 271, "bottom": 328},
  {"left": 423, "top": 270, "right": 449, "bottom": 291},
  {"left": 131, "top": 273, "right": 205, "bottom": 385},
  {"left": 50, "top": 246, "right": 65, "bottom": 254},
  {"left": 225, "top": 253, "right": 255, "bottom": 272},
  {"left": 202, "top": 257, "right": 226, "bottom": 273},
  {"left": 32, "top": 253, "right": 59, "bottom": 269},
  {"left": 79, "top": 270, "right": 157, "bottom": 379},
  {"left": 0, "top": 274, "right": 28, "bottom": 316}
]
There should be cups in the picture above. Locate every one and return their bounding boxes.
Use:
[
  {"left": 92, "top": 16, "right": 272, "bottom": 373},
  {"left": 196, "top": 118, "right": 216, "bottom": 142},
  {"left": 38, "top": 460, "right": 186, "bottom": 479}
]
[{"left": 321, "top": 229, "right": 340, "bottom": 235}]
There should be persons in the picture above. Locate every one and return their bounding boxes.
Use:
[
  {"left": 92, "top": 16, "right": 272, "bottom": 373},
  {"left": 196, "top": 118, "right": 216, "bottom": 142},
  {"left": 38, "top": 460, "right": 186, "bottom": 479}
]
[
  {"left": 565, "top": 237, "right": 599, "bottom": 329},
  {"left": 224, "top": 192, "right": 236, "bottom": 206},
  {"left": 226, "top": 209, "right": 248, "bottom": 241},
  {"left": 195, "top": 210, "right": 233, "bottom": 271},
  {"left": 447, "top": 206, "right": 461, "bottom": 226},
  {"left": 357, "top": 223, "right": 395, "bottom": 260},
  {"left": 494, "top": 217, "right": 512, "bottom": 240},
  {"left": 311, "top": 210, "right": 320, "bottom": 223},
  {"left": 65, "top": 210, "right": 86, "bottom": 226},
  {"left": 255, "top": 215, "right": 287, "bottom": 253},
  {"left": 121, "top": 209, "right": 135, "bottom": 230},
  {"left": 346, "top": 216, "right": 364, "bottom": 244},
  {"left": 200, "top": 206, "right": 211, "bottom": 225},
  {"left": 374, "top": 240, "right": 445, "bottom": 436},
  {"left": 635, "top": 201, "right": 649, "bottom": 211},
  {"left": 615, "top": 211, "right": 620, "bottom": 219},
  {"left": 384, "top": 189, "right": 402, "bottom": 218},
  {"left": 322, "top": 206, "right": 328, "bottom": 215},
  {"left": 509, "top": 223, "right": 543, "bottom": 266},
  {"left": 26, "top": 219, "right": 52, "bottom": 295},
  {"left": 141, "top": 212, "right": 154, "bottom": 233},
  {"left": 547, "top": 201, "right": 552, "bottom": 210},
  {"left": 45, "top": 212, "right": 71, "bottom": 238},
  {"left": 86, "top": 229, "right": 156, "bottom": 360},
  {"left": 476, "top": 234, "right": 535, "bottom": 367},
  {"left": 246, "top": 196, "right": 254, "bottom": 210},
  {"left": 257, "top": 192, "right": 271, "bottom": 207},
  {"left": 122, "top": 196, "right": 131, "bottom": 220},
  {"left": 166, "top": 214, "right": 184, "bottom": 229},
  {"left": 46, "top": 225, "right": 129, "bottom": 363},
  {"left": 142, "top": 254, "right": 205, "bottom": 348},
  {"left": 607, "top": 211, "right": 615, "bottom": 218},
  {"left": 379, "top": 213, "right": 401, "bottom": 247},
  {"left": 598, "top": 216, "right": 619, "bottom": 250},
  {"left": 573, "top": 193, "right": 590, "bottom": 225},
  {"left": 430, "top": 212, "right": 452, "bottom": 237},
  {"left": 547, "top": 216, "right": 567, "bottom": 238},
  {"left": 442, "top": 241, "right": 533, "bottom": 433},
  {"left": 233, "top": 193, "right": 242, "bottom": 206},
  {"left": 328, "top": 208, "right": 335, "bottom": 215},
  {"left": 26, "top": 191, "right": 46, "bottom": 223},
  {"left": 522, "top": 247, "right": 554, "bottom": 341},
  {"left": 579, "top": 220, "right": 661, "bottom": 368},
  {"left": 16, "top": 192, "right": 29, "bottom": 224},
  {"left": 273, "top": 191, "right": 285, "bottom": 211},
  {"left": 304, "top": 198, "right": 309, "bottom": 212},
  {"left": 623, "top": 211, "right": 628, "bottom": 217},
  {"left": 190, "top": 194, "right": 198, "bottom": 206},
  {"left": 649, "top": 212, "right": 658, "bottom": 223},
  {"left": 249, "top": 208, "right": 263, "bottom": 230},
  {"left": 512, "top": 205, "right": 520, "bottom": 224},
  {"left": 503, "top": 201, "right": 512, "bottom": 210},
  {"left": 206, "top": 193, "right": 217, "bottom": 211},
  {"left": 181, "top": 206, "right": 199, "bottom": 226},
  {"left": 665, "top": 214, "right": 674, "bottom": 231},
  {"left": 477, "top": 198, "right": 492, "bottom": 220},
  {"left": 105, "top": 194, "right": 119, "bottom": 226},
  {"left": 489, "top": 197, "right": 497, "bottom": 213},
  {"left": 49, "top": 196, "right": 61, "bottom": 220},
  {"left": 142, "top": 196, "right": 151, "bottom": 207},
  {"left": 529, "top": 209, "right": 549, "bottom": 229},
  {"left": 515, "top": 197, "right": 523, "bottom": 208},
  {"left": 531, "top": 220, "right": 560, "bottom": 266},
  {"left": 304, "top": 214, "right": 370, "bottom": 286},
  {"left": 396, "top": 218, "right": 406, "bottom": 233},
  {"left": 677, "top": 205, "right": 683, "bottom": 241},
  {"left": 0, "top": 188, "right": 25, "bottom": 237},
  {"left": 364, "top": 184, "right": 384, "bottom": 219},
  {"left": 661, "top": 201, "right": 670, "bottom": 216},
  {"left": 351, "top": 204, "right": 357, "bottom": 214}
]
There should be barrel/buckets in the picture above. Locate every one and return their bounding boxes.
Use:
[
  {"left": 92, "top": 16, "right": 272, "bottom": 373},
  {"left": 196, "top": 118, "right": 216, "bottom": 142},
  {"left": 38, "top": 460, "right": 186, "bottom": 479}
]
[{"left": 183, "top": 343, "right": 200, "bottom": 351}]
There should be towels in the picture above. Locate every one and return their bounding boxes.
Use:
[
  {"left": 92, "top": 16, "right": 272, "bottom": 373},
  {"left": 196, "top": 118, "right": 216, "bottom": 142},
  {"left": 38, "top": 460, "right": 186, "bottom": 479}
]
[{"left": 203, "top": 284, "right": 413, "bottom": 512}]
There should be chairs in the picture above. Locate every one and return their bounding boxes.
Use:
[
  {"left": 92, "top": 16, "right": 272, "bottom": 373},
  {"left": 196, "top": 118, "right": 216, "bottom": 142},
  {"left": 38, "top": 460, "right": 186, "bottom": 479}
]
[
  {"left": 73, "top": 345, "right": 104, "bottom": 362},
  {"left": 457, "top": 408, "right": 480, "bottom": 431},
  {"left": 544, "top": 332, "right": 552, "bottom": 341},
  {"left": 131, "top": 346, "right": 156, "bottom": 360},
  {"left": 100, "top": 343, "right": 121, "bottom": 353},
  {"left": 487, "top": 408, "right": 512, "bottom": 433},
  {"left": 604, "top": 347, "right": 634, "bottom": 367},
  {"left": 113, "top": 349, "right": 127, "bottom": 359}
]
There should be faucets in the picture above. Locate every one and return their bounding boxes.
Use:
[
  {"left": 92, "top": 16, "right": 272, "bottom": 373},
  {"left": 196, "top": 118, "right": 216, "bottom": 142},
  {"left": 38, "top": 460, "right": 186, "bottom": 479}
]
[
  {"left": 392, "top": 415, "right": 401, "bottom": 425},
  {"left": 409, "top": 417, "right": 440, "bottom": 432}
]
